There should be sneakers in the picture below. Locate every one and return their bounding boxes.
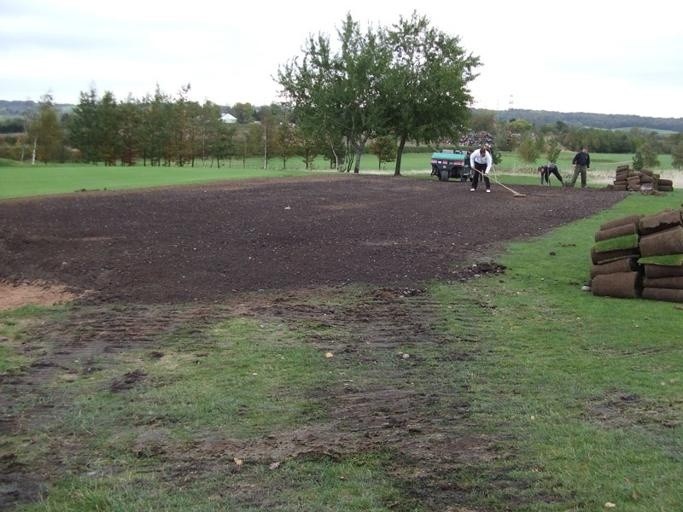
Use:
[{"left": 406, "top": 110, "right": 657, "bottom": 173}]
[
  {"left": 470, "top": 188, "right": 475, "bottom": 192},
  {"left": 486, "top": 189, "right": 491, "bottom": 193}
]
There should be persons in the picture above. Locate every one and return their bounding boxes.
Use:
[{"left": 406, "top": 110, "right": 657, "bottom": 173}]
[
  {"left": 570, "top": 145, "right": 590, "bottom": 188},
  {"left": 537, "top": 163, "right": 565, "bottom": 187},
  {"left": 469, "top": 146, "right": 492, "bottom": 193}
]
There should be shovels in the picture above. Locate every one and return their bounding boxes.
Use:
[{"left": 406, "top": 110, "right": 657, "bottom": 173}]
[{"left": 474, "top": 168, "right": 526, "bottom": 197}]
